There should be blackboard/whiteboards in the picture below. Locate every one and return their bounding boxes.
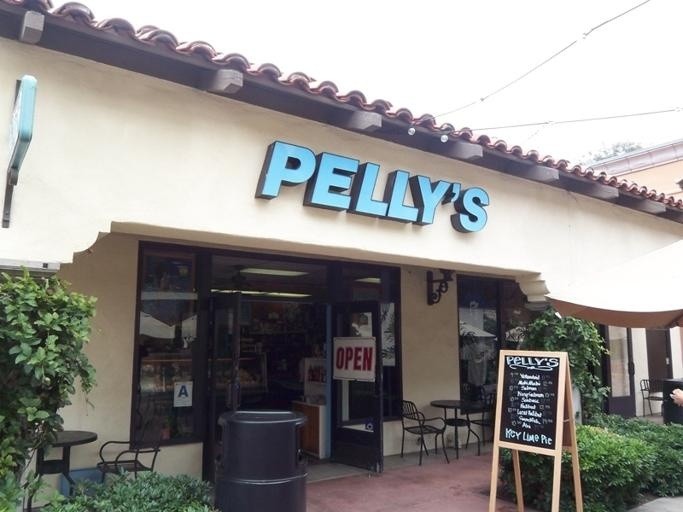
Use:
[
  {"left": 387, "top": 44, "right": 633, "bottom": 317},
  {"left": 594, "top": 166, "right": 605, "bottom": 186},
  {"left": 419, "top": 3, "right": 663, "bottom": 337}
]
[{"left": 494, "top": 350, "right": 567, "bottom": 456}]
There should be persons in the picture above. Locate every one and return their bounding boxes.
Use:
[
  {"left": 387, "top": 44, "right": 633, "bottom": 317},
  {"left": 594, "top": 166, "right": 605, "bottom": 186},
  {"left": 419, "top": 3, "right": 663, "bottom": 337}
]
[{"left": 670, "top": 387, "right": 683, "bottom": 409}]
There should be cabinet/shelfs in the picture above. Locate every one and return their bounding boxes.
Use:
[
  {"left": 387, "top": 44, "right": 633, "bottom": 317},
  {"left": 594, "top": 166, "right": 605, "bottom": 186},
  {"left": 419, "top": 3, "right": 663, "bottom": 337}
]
[{"left": 134, "top": 351, "right": 268, "bottom": 445}]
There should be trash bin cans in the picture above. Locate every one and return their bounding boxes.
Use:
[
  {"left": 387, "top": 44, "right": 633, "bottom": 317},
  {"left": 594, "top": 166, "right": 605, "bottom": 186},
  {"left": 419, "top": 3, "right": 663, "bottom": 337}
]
[{"left": 213, "top": 410, "right": 308, "bottom": 512}]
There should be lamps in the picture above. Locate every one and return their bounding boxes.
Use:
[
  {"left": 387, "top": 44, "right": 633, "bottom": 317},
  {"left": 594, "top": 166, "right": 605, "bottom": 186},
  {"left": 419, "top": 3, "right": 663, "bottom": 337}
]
[
  {"left": 426, "top": 269, "right": 455, "bottom": 306},
  {"left": 407, "top": 121, "right": 417, "bottom": 136},
  {"left": 439, "top": 131, "right": 450, "bottom": 142}
]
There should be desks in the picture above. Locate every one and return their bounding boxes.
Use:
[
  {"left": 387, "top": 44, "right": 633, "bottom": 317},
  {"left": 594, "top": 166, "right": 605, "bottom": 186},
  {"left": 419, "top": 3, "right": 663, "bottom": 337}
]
[
  {"left": 430, "top": 400, "right": 480, "bottom": 459},
  {"left": 22, "top": 431, "right": 98, "bottom": 512}
]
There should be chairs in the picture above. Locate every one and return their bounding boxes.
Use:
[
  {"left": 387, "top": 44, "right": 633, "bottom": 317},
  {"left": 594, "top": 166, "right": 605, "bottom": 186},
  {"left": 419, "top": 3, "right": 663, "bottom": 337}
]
[
  {"left": 640, "top": 379, "right": 663, "bottom": 417},
  {"left": 463, "top": 393, "right": 497, "bottom": 455},
  {"left": 98, "top": 419, "right": 165, "bottom": 483},
  {"left": 400, "top": 400, "right": 449, "bottom": 465}
]
